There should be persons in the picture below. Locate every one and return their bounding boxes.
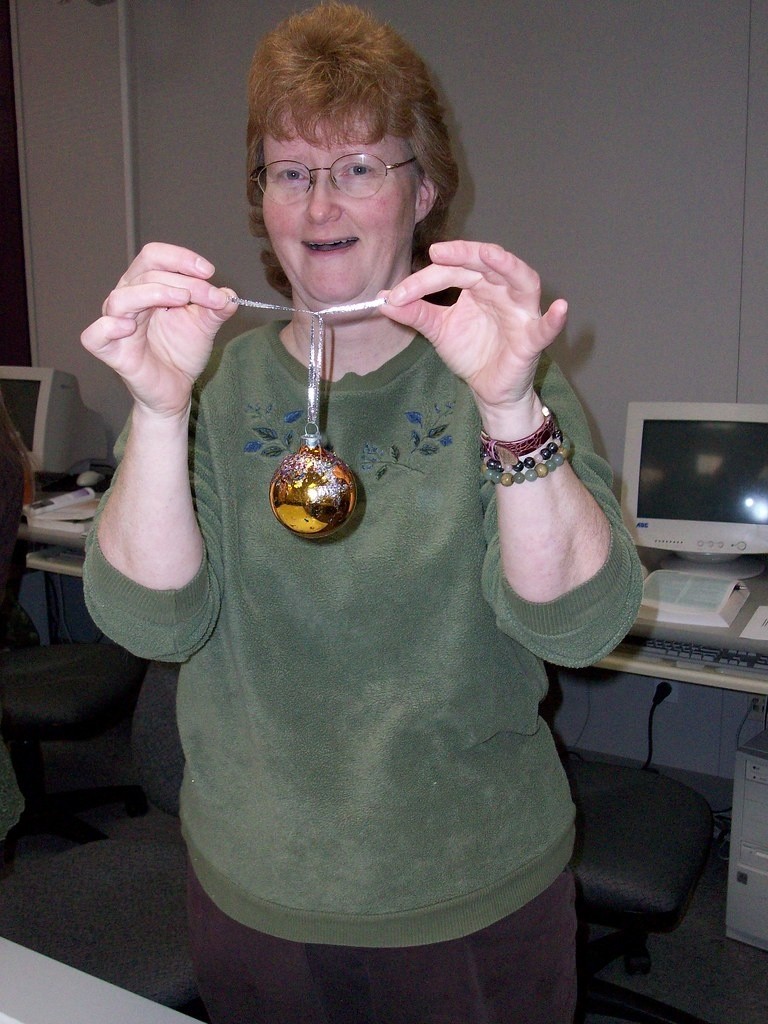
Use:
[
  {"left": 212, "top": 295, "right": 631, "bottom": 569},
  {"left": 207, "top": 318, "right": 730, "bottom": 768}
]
[{"left": 83, "top": 5, "right": 643, "bottom": 1024}]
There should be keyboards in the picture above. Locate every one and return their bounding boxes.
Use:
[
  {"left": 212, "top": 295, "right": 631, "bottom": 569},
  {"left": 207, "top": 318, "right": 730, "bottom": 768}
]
[
  {"left": 26, "top": 546, "right": 87, "bottom": 576},
  {"left": 594, "top": 634, "right": 768, "bottom": 697}
]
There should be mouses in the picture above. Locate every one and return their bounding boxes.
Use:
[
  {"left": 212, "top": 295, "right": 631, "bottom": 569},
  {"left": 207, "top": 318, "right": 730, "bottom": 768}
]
[{"left": 76, "top": 471, "right": 105, "bottom": 486}]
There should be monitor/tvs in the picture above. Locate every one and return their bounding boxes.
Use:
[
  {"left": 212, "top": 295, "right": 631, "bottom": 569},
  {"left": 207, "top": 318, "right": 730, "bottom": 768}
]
[
  {"left": 0, "top": 365, "right": 109, "bottom": 476},
  {"left": 620, "top": 401, "right": 768, "bottom": 580}
]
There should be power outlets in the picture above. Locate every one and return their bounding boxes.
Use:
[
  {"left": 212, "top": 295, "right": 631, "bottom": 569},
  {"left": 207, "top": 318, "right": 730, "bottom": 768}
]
[
  {"left": 747, "top": 695, "right": 767, "bottom": 723},
  {"left": 653, "top": 681, "right": 677, "bottom": 703}
]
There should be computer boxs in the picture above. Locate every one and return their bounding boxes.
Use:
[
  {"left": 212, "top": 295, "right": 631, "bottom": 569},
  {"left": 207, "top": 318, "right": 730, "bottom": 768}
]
[{"left": 726, "top": 729, "right": 768, "bottom": 952}]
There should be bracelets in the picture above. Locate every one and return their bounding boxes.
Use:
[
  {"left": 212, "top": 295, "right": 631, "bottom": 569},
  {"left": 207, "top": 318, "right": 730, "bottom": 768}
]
[{"left": 476, "top": 399, "right": 575, "bottom": 487}]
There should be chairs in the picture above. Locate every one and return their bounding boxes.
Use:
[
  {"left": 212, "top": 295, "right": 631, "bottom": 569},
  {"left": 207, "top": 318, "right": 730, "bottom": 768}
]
[{"left": 1, "top": 542, "right": 718, "bottom": 1024}]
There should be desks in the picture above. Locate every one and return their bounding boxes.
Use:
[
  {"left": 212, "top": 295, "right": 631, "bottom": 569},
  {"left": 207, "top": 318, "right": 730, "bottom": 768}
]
[{"left": 18, "top": 488, "right": 768, "bottom": 696}]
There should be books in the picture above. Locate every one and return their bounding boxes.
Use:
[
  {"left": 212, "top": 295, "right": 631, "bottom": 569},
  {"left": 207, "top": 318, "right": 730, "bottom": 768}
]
[
  {"left": 23, "top": 485, "right": 102, "bottom": 533},
  {"left": 634, "top": 562, "right": 750, "bottom": 628}
]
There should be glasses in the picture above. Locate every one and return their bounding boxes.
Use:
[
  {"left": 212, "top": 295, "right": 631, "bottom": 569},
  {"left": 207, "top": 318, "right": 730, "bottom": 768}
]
[{"left": 250, "top": 152, "right": 416, "bottom": 205}]
